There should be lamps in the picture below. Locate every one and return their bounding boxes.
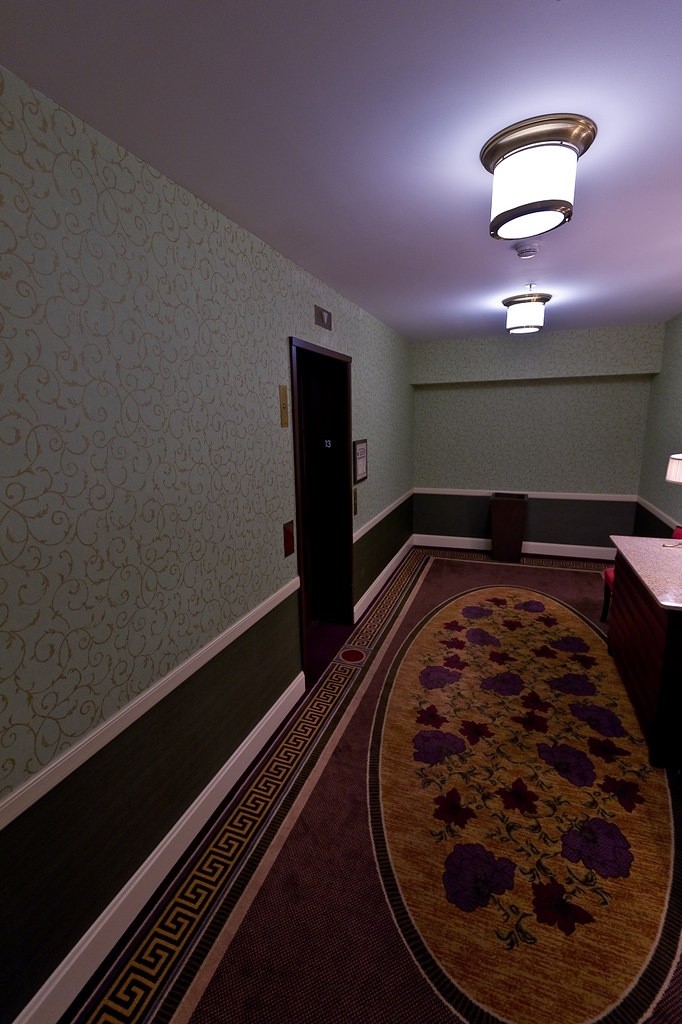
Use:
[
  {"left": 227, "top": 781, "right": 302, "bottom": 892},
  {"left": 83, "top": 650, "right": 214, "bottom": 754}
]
[
  {"left": 502, "top": 293, "right": 552, "bottom": 334},
  {"left": 480, "top": 112, "right": 598, "bottom": 240},
  {"left": 665, "top": 453, "right": 682, "bottom": 486}
]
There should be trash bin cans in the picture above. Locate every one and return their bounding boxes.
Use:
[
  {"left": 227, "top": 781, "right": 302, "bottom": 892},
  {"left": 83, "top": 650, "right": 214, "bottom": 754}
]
[{"left": 489, "top": 492, "right": 529, "bottom": 564}]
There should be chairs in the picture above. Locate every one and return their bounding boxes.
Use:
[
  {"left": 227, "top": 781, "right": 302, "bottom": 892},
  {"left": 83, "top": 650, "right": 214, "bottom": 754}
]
[{"left": 600, "top": 525, "right": 682, "bottom": 622}]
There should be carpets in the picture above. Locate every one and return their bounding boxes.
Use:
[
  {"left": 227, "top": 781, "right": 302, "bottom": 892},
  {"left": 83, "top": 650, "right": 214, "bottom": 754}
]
[{"left": 54, "top": 545, "right": 682, "bottom": 1024}]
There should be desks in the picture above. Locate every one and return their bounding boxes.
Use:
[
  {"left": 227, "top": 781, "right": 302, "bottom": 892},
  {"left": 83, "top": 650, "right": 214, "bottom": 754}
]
[{"left": 606, "top": 534, "right": 682, "bottom": 768}]
